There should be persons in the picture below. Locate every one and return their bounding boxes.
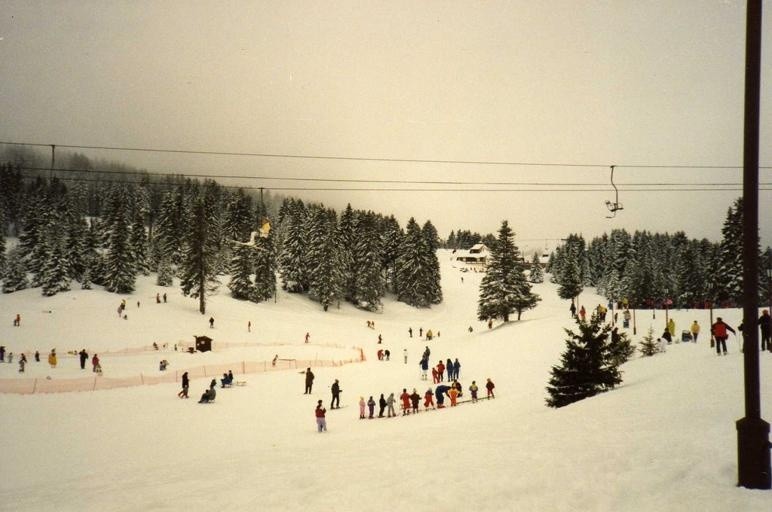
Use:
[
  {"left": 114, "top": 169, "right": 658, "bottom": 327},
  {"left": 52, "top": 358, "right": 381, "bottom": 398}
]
[
  {"left": 303, "top": 367, "right": 314, "bottom": 394},
  {"left": 157, "top": 292, "right": 167, "bottom": 303},
  {"left": 117, "top": 299, "right": 127, "bottom": 319},
  {"left": 643, "top": 297, "right": 733, "bottom": 309},
  {"left": 209, "top": 316, "right": 214, "bottom": 328},
  {"left": 248, "top": 321, "right": 251, "bottom": 333},
  {"left": 272, "top": 354, "right": 278, "bottom": 366},
  {"left": 358, "top": 319, "right": 496, "bottom": 420},
  {"left": 568, "top": 302, "right": 700, "bottom": 353},
  {"left": 14, "top": 314, "right": 21, "bottom": 326},
  {"left": 152, "top": 341, "right": 233, "bottom": 403},
  {"left": 137, "top": 300, "right": 140, "bottom": 308},
  {"left": 710, "top": 317, "right": 736, "bottom": 355},
  {"left": 0, "top": 345, "right": 100, "bottom": 373},
  {"left": 305, "top": 332, "right": 310, "bottom": 343},
  {"left": 316, "top": 400, "right": 327, "bottom": 433},
  {"left": 330, "top": 379, "right": 343, "bottom": 409},
  {"left": 737, "top": 309, "right": 772, "bottom": 353}
]
